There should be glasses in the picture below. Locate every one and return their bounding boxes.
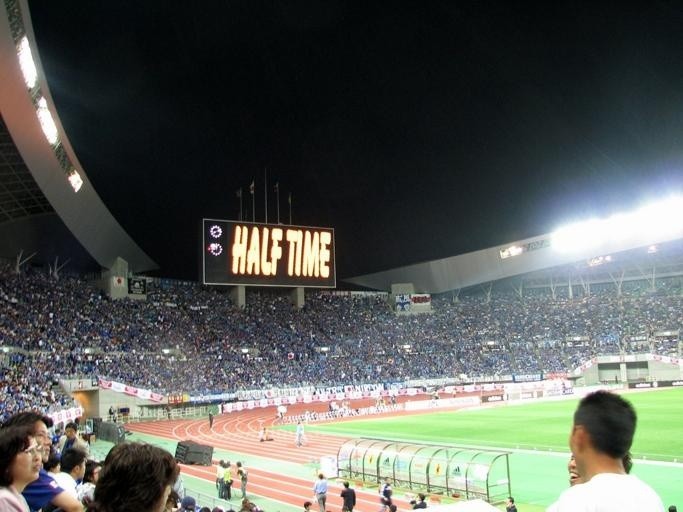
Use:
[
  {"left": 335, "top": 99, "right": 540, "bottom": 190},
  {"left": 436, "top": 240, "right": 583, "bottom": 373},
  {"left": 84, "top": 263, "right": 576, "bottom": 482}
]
[{"left": 21, "top": 445, "right": 47, "bottom": 456}]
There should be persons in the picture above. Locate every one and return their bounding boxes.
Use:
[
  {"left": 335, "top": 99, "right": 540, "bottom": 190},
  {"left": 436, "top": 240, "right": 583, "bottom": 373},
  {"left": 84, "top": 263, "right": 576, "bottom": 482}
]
[
  {"left": 44, "top": 432, "right": 264, "bottom": 512},
  {"left": 339, "top": 482, "right": 356, "bottom": 512},
  {"left": 295, "top": 422, "right": 304, "bottom": 449},
  {"left": 504, "top": 497, "right": 517, "bottom": 512},
  {"left": 540, "top": 388, "right": 669, "bottom": 512},
  {"left": 376, "top": 477, "right": 427, "bottom": 512},
  {"left": 0, "top": 408, "right": 84, "bottom": 512},
  {"left": 304, "top": 502, "right": 312, "bottom": 512},
  {"left": 566, "top": 451, "right": 632, "bottom": 488},
  {"left": 53, "top": 420, "right": 90, "bottom": 460},
  {"left": 0, "top": 425, "right": 43, "bottom": 512},
  {"left": 260, "top": 427, "right": 268, "bottom": 442},
  {"left": 207, "top": 409, "right": 214, "bottom": 431},
  {"left": 1, "top": 257, "right": 683, "bottom": 427},
  {"left": 313, "top": 473, "right": 328, "bottom": 512}
]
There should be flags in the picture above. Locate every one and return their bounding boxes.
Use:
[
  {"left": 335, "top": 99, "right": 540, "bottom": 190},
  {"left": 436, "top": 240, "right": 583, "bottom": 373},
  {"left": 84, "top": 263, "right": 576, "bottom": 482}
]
[
  {"left": 236, "top": 188, "right": 241, "bottom": 197},
  {"left": 272, "top": 182, "right": 278, "bottom": 191},
  {"left": 249, "top": 178, "right": 254, "bottom": 194},
  {"left": 287, "top": 192, "right": 291, "bottom": 205}
]
